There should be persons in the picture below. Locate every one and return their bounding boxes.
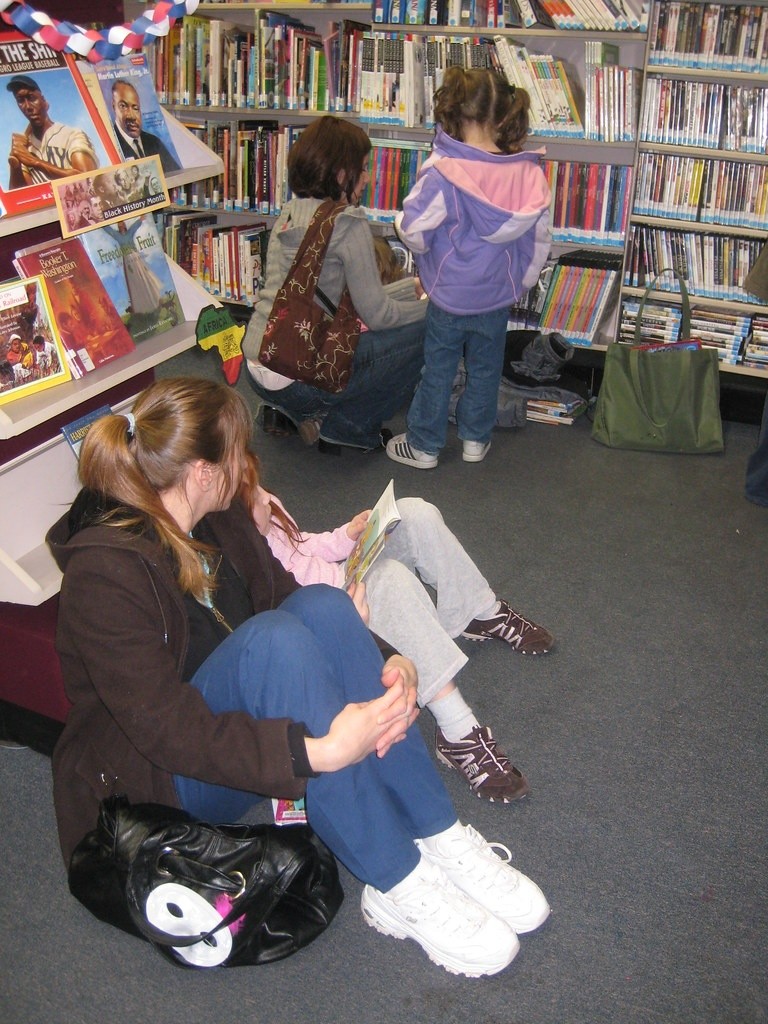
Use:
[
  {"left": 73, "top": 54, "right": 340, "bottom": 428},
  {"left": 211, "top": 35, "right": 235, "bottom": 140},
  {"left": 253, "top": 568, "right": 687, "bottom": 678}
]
[
  {"left": 0, "top": 282, "right": 62, "bottom": 393},
  {"left": 63, "top": 165, "right": 162, "bottom": 230},
  {"left": 239, "top": 115, "right": 429, "bottom": 459},
  {"left": 6, "top": 74, "right": 99, "bottom": 192},
  {"left": 382, "top": 66, "right": 552, "bottom": 469},
  {"left": 232, "top": 449, "right": 562, "bottom": 804},
  {"left": 102, "top": 215, "right": 166, "bottom": 314},
  {"left": 744, "top": 239, "right": 768, "bottom": 506},
  {"left": 110, "top": 80, "right": 180, "bottom": 175},
  {"left": 43, "top": 375, "right": 550, "bottom": 978}
]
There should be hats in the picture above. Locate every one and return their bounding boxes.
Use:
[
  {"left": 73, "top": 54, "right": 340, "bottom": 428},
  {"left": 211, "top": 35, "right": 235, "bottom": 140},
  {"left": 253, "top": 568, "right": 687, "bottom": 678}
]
[{"left": 6, "top": 75, "right": 39, "bottom": 92}]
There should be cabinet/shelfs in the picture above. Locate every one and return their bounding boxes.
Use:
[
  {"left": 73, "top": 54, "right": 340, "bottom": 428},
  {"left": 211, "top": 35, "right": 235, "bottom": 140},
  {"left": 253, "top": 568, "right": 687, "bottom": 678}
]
[{"left": 1, "top": 1, "right": 767, "bottom": 757}]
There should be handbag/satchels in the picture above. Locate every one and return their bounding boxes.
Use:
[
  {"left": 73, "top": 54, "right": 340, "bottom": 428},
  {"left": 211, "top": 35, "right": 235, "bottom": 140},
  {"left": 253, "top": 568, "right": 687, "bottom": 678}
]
[
  {"left": 68, "top": 792, "right": 344, "bottom": 967},
  {"left": 590, "top": 269, "right": 723, "bottom": 455},
  {"left": 257, "top": 200, "right": 363, "bottom": 393}
]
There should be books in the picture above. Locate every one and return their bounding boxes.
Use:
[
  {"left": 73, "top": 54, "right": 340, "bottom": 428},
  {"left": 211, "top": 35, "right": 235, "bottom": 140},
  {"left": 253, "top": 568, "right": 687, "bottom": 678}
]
[
  {"left": 140, "top": 0, "right": 768, "bottom": 425},
  {"left": 0, "top": 276, "right": 72, "bottom": 405},
  {"left": 340, "top": 478, "right": 402, "bottom": 593},
  {"left": 77, "top": 212, "right": 185, "bottom": 344},
  {"left": 60, "top": 404, "right": 115, "bottom": 461},
  {"left": 14, "top": 236, "right": 137, "bottom": 380},
  {"left": 0, "top": 30, "right": 184, "bottom": 218}
]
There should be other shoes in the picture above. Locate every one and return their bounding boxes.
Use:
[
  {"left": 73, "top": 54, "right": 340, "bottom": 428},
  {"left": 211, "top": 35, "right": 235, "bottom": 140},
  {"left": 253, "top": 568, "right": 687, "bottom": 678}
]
[
  {"left": 261, "top": 403, "right": 305, "bottom": 450},
  {"left": 319, "top": 429, "right": 392, "bottom": 454}
]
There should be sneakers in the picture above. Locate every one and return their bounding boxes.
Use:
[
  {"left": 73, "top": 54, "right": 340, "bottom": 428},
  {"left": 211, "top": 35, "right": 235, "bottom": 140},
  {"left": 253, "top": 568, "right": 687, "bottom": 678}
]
[
  {"left": 387, "top": 432, "right": 438, "bottom": 469},
  {"left": 461, "top": 599, "right": 554, "bottom": 654},
  {"left": 462, "top": 440, "right": 491, "bottom": 462},
  {"left": 436, "top": 724, "right": 529, "bottom": 803},
  {"left": 421, "top": 823, "right": 551, "bottom": 933},
  {"left": 361, "top": 849, "right": 522, "bottom": 977}
]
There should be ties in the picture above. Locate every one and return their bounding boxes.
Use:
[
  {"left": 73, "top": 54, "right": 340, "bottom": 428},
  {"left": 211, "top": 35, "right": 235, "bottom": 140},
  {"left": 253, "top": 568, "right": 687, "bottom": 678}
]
[{"left": 133, "top": 139, "right": 144, "bottom": 158}]
